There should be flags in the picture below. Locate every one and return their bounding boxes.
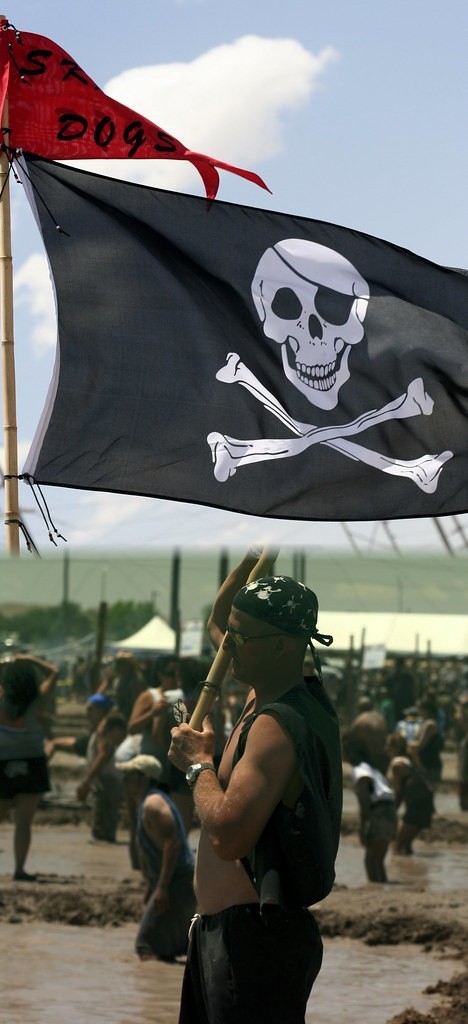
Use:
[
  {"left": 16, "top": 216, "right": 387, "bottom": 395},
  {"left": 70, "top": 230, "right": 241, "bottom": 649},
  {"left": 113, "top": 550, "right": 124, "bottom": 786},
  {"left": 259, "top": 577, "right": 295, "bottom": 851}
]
[{"left": 14, "top": 150, "right": 467, "bottom": 522}]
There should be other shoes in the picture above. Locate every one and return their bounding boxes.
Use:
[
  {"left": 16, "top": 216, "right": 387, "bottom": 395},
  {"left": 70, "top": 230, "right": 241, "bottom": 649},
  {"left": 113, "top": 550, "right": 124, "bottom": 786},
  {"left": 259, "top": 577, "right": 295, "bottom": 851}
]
[{"left": 12, "top": 868, "right": 36, "bottom": 883}]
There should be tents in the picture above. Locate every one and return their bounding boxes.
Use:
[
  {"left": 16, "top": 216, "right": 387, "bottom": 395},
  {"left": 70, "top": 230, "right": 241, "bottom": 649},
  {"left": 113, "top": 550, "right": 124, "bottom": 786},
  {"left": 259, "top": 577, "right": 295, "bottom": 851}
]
[
  {"left": 308, "top": 612, "right": 468, "bottom": 658},
  {"left": 114, "top": 615, "right": 192, "bottom": 660}
]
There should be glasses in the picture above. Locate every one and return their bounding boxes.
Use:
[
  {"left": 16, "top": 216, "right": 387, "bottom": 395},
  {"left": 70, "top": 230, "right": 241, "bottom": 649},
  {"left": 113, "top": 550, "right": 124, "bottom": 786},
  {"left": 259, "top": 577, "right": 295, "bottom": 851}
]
[{"left": 224, "top": 626, "right": 296, "bottom": 646}]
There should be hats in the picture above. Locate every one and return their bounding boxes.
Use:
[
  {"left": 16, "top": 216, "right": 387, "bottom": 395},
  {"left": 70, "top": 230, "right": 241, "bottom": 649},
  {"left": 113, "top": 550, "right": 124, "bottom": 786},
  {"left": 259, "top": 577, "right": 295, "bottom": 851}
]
[
  {"left": 89, "top": 694, "right": 115, "bottom": 712},
  {"left": 114, "top": 754, "right": 163, "bottom": 782},
  {"left": 232, "top": 575, "right": 334, "bottom": 686}
]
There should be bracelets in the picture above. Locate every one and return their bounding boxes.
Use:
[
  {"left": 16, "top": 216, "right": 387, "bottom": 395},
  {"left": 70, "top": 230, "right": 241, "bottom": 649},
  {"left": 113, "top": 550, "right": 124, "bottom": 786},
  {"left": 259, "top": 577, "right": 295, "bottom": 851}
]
[{"left": 249, "top": 546, "right": 258, "bottom": 558}]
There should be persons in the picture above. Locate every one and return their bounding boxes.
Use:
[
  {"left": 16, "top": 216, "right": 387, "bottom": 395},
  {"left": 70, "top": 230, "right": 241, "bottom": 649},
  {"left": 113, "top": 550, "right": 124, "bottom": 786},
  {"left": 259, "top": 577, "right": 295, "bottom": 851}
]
[{"left": 1, "top": 518, "right": 468, "bottom": 1024}]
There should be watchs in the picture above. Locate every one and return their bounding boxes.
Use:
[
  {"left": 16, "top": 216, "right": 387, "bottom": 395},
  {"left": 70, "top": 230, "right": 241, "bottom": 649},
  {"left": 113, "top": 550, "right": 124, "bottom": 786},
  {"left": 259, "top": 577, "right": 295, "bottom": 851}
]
[{"left": 185, "top": 763, "right": 215, "bottom": 791}]
